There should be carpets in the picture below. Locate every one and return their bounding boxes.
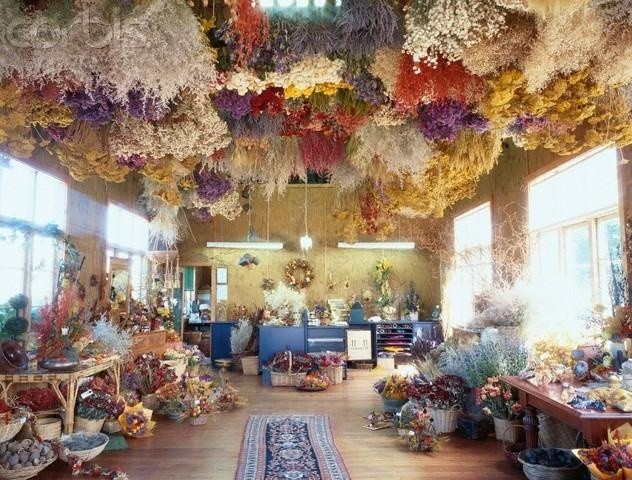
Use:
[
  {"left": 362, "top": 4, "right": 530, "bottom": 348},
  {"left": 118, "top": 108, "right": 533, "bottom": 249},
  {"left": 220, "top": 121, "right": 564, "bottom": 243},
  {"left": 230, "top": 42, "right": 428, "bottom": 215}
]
[{"left": 231, "top": 410, "right": 354, "bottom": 480}]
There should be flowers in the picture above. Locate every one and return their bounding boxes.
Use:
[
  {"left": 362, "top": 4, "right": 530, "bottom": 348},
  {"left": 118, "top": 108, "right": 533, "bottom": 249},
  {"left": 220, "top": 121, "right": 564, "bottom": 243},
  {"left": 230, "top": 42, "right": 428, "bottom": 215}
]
[
  {"left": 350, "top": 252, "right": 632, "bottom": 479},
  {"left": 2, "top": 253, "right": 256, "bottom": 480},
  {"left": 282, "top": 257, "right": 317, "bottom": 290},
  {"left": 0, "top": 0, "right": 631, "bottom": 252},
  {"left": 260, "top": 347, "right": 348, "bottom": 392}
]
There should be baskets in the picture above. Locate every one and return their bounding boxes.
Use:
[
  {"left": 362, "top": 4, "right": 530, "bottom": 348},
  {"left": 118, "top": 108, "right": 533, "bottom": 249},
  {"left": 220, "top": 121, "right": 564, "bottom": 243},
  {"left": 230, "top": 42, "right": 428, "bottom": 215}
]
[
  {"left": 493, "top": 409, "right": 584, "bottom": 480},
  {"left": 452, "top": 326, "right": 520, "bottom": 344},
  {"left": 318, "top": 366, "right": 343, "bottom": 385},
  {"left": 142, "top": 394, "right": 160, "bottom": 409},
  {"left": 0, "top": 410, "right": 110, "bottom": 480},
  {"left": 102, "top": 407, "right": 153, "bottom": 433},
  {"left": 426, "top": 403, "right": 462, "bottom": 433},
  {"left": 271, "top": 351, "right": 307, "bottom": 387},
  {"left": 229, "top": 352, "right": 259, "bottom": 375}
]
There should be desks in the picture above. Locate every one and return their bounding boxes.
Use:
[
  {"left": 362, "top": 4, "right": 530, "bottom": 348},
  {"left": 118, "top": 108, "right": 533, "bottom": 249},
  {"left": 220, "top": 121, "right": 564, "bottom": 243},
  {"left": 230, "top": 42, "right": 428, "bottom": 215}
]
[{"left": 257, "top": 323, "right": 350, "bottom": 382}]
